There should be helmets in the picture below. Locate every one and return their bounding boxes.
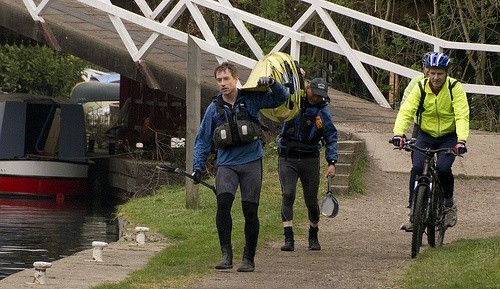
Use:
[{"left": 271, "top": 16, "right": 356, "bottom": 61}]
[
  {"left": 426, "top": 52, "right": 451, "bottom": 69},
  {"left": 423, "top": 52, "right": 435, "bottom": 65}
]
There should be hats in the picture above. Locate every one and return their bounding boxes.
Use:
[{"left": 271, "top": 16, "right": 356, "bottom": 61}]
[{"left": 310, "top": 78, "right": 329, "bottom": 98}]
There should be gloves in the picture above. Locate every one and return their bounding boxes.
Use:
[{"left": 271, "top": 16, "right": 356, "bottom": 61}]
[
  {"left": 454, "top": 143, "right": 467, "bottom": 154},
  {"left": 258, "top": 76, "right": 274, "bottom": 87},
  {"left": 392, "top": 135, "right": 407, "bottom": 150},
  {"left": 189, "top": 172, "right": 203, "bottom": 184}
]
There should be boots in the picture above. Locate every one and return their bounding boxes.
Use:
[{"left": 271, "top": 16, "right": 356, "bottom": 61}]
[
  {"left": 239, "top": 246, "right": 256, "bottom": 271},
  {"left": 216, "top": 246, "right": 234, "bottom": 268}
]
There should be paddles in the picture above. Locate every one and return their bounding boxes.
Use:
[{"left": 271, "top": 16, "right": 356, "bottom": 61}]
[
  {"left": 156, "top": 165, "right": 216, "bottom": 194},
  {"left": 320, "top": 176, "right": 339, "bottom": 218}
]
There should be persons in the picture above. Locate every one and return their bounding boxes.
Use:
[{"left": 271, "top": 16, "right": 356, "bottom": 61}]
[
  {"left": 401, "top": 53, "right": 430, "bottom": 207},
  {"left": 392, "top": 52, "right": 469, "bottom": 231},
  {"left": 278, "top": 68, "right": 338, "bottom": 251},
  {"left": 191, "top": 63, "right": 289, "bottom": 272}
]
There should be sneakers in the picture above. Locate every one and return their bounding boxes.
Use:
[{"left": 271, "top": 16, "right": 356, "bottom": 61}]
[
  {"left": 444, "top": 202, "right": 458, "bottom": 227},
  {"left": 281, "top": 238, "right": 294, "bottom": 251},
  {"left": 401, "top": 214, "right": 414, "bottom": 230},
  {"left": 308, "top": 226, "right": 321, "bottom": 251}
]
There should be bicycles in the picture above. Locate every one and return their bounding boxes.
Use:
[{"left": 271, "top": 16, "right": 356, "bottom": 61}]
[{"left": 390, "top": 136, "right": 468, "bottom": 260}]
